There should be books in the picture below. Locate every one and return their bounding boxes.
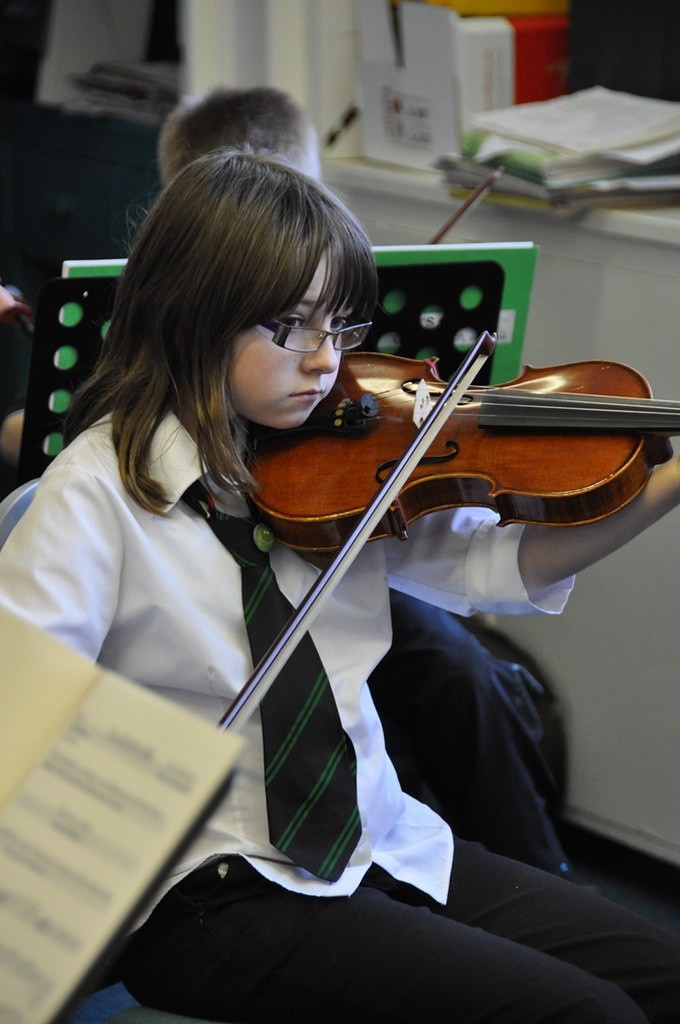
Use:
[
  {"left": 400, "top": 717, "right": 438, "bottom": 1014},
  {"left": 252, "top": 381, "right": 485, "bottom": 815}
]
[
  {"left": 430, "top": 76, "right": 679, "bottom": 225},
  {"left": 76, "top": 59, "right": 180, "bottom": 124}
]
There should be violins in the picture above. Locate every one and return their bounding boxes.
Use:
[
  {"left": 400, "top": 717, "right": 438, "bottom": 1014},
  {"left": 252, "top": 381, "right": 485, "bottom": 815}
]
[{"left": 239, "top": 347, "right": 680, "bottom": 548}]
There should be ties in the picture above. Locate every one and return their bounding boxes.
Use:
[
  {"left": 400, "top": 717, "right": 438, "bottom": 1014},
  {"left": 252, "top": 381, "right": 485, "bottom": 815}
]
[{"left": 181, "top": 478, "right": 363, "bottom": 885}]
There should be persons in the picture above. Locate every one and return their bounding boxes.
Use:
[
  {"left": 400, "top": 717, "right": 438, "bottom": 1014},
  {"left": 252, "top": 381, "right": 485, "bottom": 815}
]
[
  {"left": 0, "top": 149, "right": 679, "bottom": 1023},
  {"left": 0, "top": 277, "right": 35, "bottom": 496},
  {"left": 157, "top": 84, "right": 581, "bottom": 884}
]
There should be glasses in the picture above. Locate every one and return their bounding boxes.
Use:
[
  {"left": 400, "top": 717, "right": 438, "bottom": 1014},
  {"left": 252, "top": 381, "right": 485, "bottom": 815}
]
[{"left": 259, "top": 316, "right": 375, "bottom": 353}]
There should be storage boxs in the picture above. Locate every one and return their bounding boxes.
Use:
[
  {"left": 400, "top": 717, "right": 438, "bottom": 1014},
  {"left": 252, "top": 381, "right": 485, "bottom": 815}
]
[{"left": 356, "top": 2, "right": 513, "bottom": 171}]
